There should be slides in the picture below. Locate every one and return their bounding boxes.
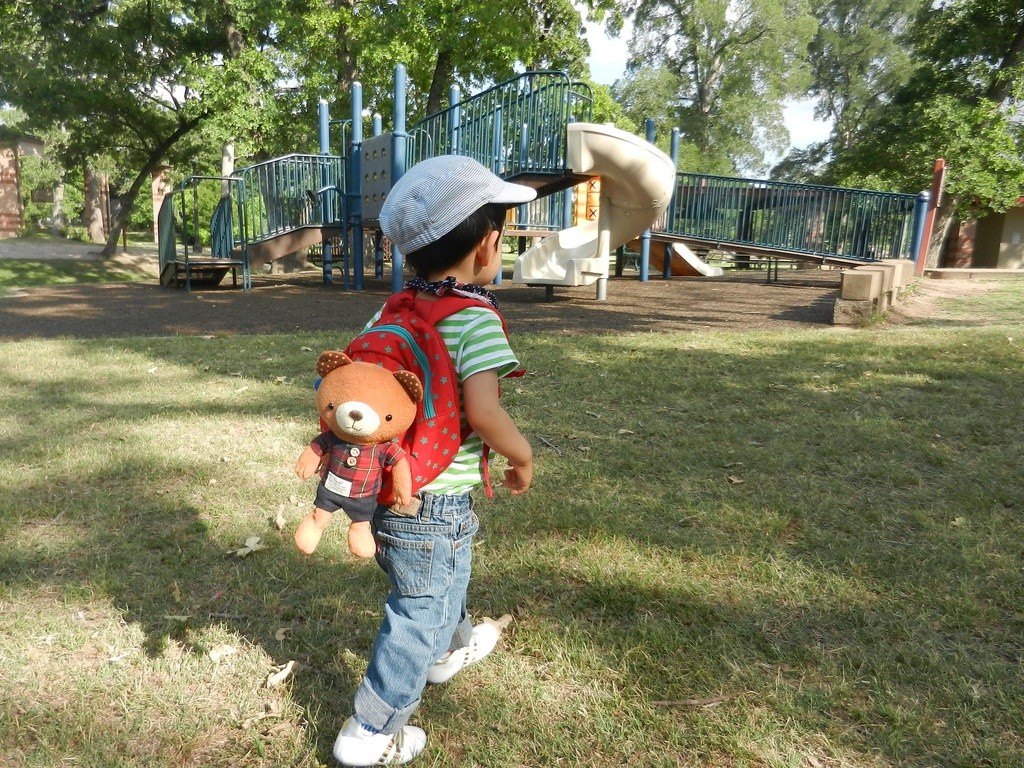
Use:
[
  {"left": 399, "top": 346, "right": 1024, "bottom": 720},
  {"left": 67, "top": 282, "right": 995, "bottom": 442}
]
[
  {"left": 514, "top": 122, "right": 676, "bottom": 287},
  {"left": 628, "top": 233, "right": 723, "bottom": 278}
]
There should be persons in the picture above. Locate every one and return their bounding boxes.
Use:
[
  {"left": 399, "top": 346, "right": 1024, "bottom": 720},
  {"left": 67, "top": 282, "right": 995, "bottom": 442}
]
[{"left": 330, "top": 154, "right": 538, "bottom": 768}]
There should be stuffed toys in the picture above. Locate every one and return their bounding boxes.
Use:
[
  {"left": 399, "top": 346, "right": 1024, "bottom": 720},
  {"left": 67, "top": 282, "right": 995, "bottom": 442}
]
[{"left": 292, "top": 351, "right": 424, "bottom": 556}]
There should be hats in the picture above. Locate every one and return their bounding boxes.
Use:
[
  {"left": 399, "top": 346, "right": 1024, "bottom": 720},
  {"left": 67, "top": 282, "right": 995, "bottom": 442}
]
[{"left": 379, "top": 155, "right": 538, "bottom": 254}]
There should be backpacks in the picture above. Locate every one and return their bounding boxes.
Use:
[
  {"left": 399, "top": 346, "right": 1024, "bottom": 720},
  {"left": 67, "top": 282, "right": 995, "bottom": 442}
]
[{"left": 320, "top": 288, "right": 527, "bottom": 506}]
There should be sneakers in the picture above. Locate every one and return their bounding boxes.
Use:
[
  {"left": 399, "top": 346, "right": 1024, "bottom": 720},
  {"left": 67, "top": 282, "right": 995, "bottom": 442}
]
[
  {"left": 427, "top": 624, "right": 498, "bottom": 683},
  {"left": 333, "top": 716, "right": 427, "bottom": 766}
]
